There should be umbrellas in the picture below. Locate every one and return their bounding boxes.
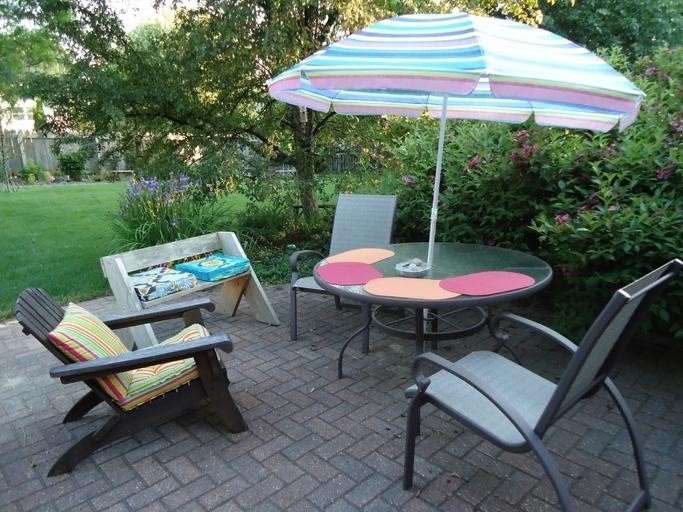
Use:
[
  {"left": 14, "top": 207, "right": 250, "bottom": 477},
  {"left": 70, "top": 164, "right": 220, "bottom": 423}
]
[{"left": 266, "top": 11, "right": 649, "bottom": 351}]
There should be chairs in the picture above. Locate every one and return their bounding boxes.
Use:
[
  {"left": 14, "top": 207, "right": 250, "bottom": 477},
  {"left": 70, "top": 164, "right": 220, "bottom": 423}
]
[
  {"left": 288, "top": 193, "right": 398, "bottom": 354},
  {"left": 402, "top": 258, "right": 682, "bottom": 512},
  {"left": 14, "top": 286, "right": 249, "bottom": 478}
]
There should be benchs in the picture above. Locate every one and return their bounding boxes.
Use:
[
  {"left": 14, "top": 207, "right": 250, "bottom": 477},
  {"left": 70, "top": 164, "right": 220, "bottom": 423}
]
[{"left": 99, "top": 230, "right": 280, "bottom": 349}]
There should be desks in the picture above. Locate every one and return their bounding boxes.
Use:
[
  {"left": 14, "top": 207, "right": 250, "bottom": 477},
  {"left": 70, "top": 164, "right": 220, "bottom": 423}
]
[{"left": 312, "top": 241, "right": 556, "bottom": 377}]
